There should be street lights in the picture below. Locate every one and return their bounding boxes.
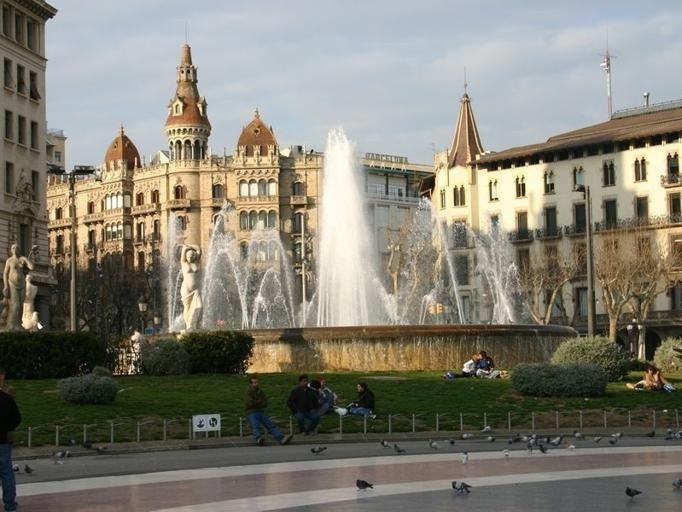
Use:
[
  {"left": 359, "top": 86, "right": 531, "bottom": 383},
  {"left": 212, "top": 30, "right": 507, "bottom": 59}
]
[
  {"left": 152, "top": 312, "right": 162, "bottom": 332},
  {"left": 627, "top": 317, "right": 644, "bottom": 359},
  {"left": 573, "top": 183, "right": 596, "bottom": 337},
  {"left": 67, "top": 174, "right": 79, "bottom": 333},
  {"left": 137, "top": 292, "right": 147, "bottom": 334}
]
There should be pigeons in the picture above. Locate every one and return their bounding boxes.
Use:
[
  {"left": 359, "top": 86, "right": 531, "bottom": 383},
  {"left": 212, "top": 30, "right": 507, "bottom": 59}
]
[
  {"left": 311, "top": 446, "right": 327, "bottom": 456},
  {"left": 94, "top": 446, "right": 107, "bottom": 455},
  {"left": 452, "top": 480, "right": 473, "bottom": 494},
  {"left": 664, "top": 427, "right": 682, "bottom": 441},
  {"left": 427, "top": 426, "right": 623, "bottom": 465},
  {"left": 51, "top": 449, "right": 72, "bottom": 464},
  {"left": 625, "top": 486, "right": 643, "bottom": 502},
  {"left": 12, "top": 464, "right": 20, "bottom": 474},
  {"left": 672, "top": 478, "right": 682, "bottom": 489},
  {"left": 355, "top": 479, "right": 374, "bottom": 492},
  {"left": 394, "top": 443, "right": 407, "bottom": 454},
  {"left": 24, "top": 464, "right": 37, "bottom": 474},
  {"left": 380, "top": 439, "right": 392, "bottom": 448},
  {"left": 646, "top": 430, "right": 656, "bottom": 438}
]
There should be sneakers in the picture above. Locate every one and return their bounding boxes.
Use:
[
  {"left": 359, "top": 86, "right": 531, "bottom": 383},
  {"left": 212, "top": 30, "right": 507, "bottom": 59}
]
[
  {"left": 257, "top": 436, "right": 265, "bottom": 445},
  {"left": 280, "top": 434, "right": 292, "bottom": 444}
]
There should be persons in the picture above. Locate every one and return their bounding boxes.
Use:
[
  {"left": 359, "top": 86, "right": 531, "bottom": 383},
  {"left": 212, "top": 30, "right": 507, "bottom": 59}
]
[
  {"left": 625, "top": 366, "right": 676, "bottom": 394},
  {"left": 178, "top": 242, "right": 201, "bottom": 329},
  {"left": 118, "top": 325, "right": 143, "bottom": 376},
  {"left": 474, "top": 351, "right": 511, "bottom": 380},
  {"left": 440, "top": 349, "right": 480, "bottom": 381},
  {"left": 2, "top": 243, "right": 37, "bottom": 328},
  {"left": 244, "top": 375, "right": 294, "bottom": 445},
  {"left": 0, "top": 365, "right": 24, "bottom": 512},
  {"left": 287, "top": 374, "right": 377, "bottom": 436}
]
